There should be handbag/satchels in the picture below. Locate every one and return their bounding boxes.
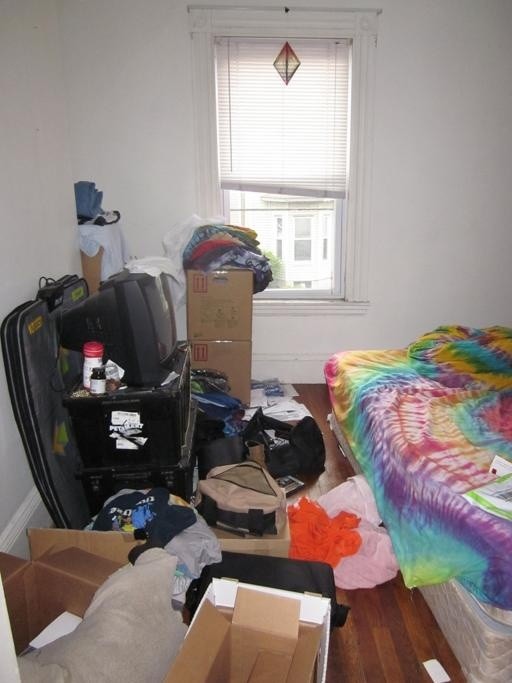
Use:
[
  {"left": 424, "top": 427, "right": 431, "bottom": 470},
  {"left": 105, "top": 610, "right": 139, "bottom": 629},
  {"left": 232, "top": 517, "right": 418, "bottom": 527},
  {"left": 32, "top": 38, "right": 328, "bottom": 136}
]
[
  {"left": 244, "top": 406, "right": 326, "bottom": 478},
  {"left": 194, "top": 459, "right": 288, "bottom": 537}
]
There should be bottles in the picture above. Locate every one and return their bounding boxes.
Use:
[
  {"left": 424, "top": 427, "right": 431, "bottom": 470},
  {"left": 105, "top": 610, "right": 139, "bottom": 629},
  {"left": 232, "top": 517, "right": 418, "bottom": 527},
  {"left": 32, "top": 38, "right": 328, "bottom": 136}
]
[{"left": 82, "top": 341, "right": 121, "bottom": 396}]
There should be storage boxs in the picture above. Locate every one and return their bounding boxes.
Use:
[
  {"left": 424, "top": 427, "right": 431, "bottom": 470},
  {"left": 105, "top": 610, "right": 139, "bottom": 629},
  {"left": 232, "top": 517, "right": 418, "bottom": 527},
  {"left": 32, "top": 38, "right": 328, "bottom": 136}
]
[
  {"left": 1, "top": 521, "right": 178, "bottom": 682},
  {"left": 178, "top": 267, "right": 253, "bottom": 420},
  {"left": 163, "top": 551, "right": 329, "bottom": 682},
  {"left": 190, "top": 492, "right": 290, "bottom": 559}
]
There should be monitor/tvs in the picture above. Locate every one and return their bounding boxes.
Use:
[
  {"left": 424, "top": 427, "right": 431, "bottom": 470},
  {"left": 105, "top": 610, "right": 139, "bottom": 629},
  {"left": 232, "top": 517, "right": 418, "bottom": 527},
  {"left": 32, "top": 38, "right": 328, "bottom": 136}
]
[{"left": 59, "top": 269, "right": 178, "bottom": 384}]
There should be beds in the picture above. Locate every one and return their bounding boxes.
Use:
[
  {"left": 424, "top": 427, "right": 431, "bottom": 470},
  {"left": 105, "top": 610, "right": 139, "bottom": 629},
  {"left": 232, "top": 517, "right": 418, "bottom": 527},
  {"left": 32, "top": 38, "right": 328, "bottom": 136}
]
[{"left": 326, "top": 344, "right": 512, "bottom": 679}]
